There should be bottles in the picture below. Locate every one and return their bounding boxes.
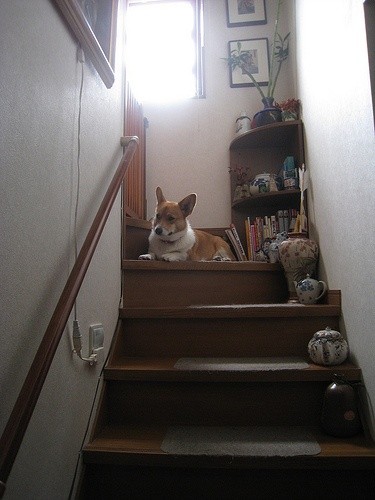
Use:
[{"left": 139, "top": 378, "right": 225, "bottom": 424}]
[
  {"left": 236, "top": 112, "right": 251, "bottom": 135},
  {"left": 321, "top": 373, "right": 360, "bottom": 437}
]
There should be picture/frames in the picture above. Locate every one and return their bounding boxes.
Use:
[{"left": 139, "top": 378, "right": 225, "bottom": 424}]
[
  {"left": 55, "top": 0, "right": 119, "bottom": 90},
  {"left": 228, "top": 37, "right": 273, "bottom": 88},
  {"left": 225, "top": 0, "right": 267, "bottom": 27}
]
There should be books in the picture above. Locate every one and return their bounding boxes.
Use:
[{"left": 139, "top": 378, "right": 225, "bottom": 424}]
[{"left": 224, "top": 208, "right": 300, "bottom": 262}]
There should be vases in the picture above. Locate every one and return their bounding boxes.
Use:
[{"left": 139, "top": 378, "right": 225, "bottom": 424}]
[{"left": 278, "top": 233, "right": 319, "bottom": 303}]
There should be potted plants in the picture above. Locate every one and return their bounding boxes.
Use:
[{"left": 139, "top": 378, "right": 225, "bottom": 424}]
[{"left": 221, "top": 0, "right": 292, "bottom": 126}]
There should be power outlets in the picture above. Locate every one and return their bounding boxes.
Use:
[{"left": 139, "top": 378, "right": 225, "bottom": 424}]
[{"left": 88, "top": 324, "right": 105, "bottom": 365}]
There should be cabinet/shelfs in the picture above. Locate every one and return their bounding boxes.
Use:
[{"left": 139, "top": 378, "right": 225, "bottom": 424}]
[{"left": 229, "top": 120, "right": 308, "bottom": 260}]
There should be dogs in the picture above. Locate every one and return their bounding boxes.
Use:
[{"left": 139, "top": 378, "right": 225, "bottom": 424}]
[{"left": 139, "top": 187, "right": 236, "bottom": 262}]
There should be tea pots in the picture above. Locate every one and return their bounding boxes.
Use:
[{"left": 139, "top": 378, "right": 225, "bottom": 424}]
[{"left": 293, "top": 274, "right": 327, "bottom": 304}]
